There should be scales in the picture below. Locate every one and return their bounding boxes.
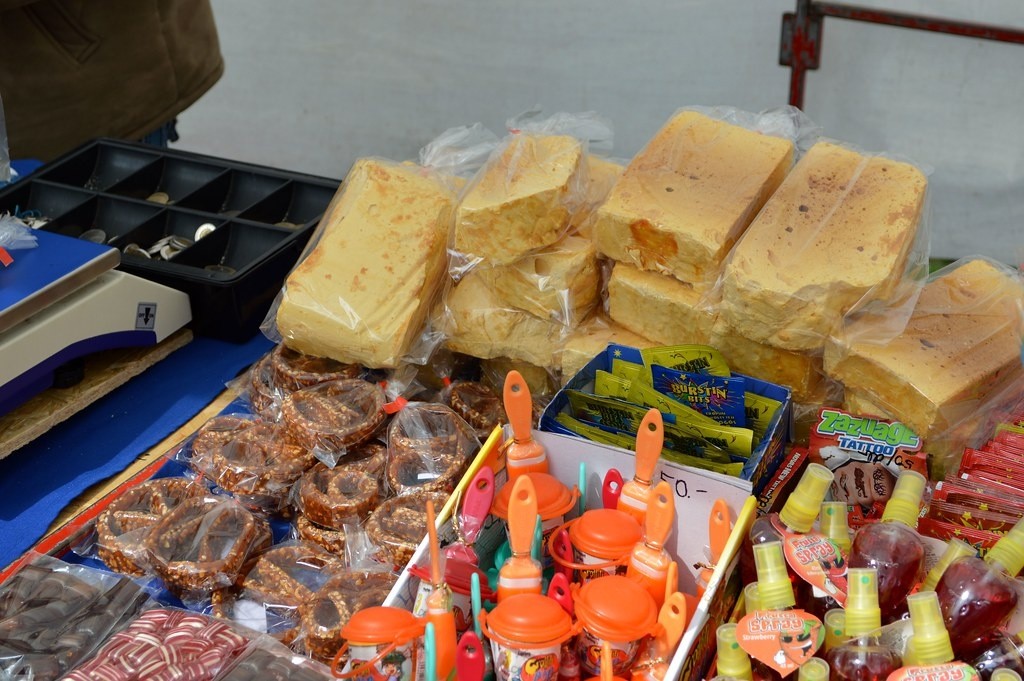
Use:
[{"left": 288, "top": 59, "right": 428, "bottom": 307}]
[{"left": 0, "top": 218, "right": 193, "bottom": 424}]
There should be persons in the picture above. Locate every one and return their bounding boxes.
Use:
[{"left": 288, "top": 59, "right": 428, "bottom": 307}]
[{"left": 0, "top": 0, "right": 224, "bottom": 160}]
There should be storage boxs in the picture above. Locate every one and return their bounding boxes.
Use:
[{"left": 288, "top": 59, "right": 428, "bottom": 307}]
[
  {"left": 336, "top": 423, "right": 757, "bottom": 681},
  {"left": 0, "top": 137, "right": 342, "bottom": 343},
  {"left": 755, "top": 445, "right": 810, "bottom": 517},
  {"left": 539, "top": 342, "right": 792, "bottom": 495}
]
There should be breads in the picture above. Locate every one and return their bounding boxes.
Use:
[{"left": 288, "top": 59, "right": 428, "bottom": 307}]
[{"left": 277, "top": 110, "right": 1024, "bottom": 476}]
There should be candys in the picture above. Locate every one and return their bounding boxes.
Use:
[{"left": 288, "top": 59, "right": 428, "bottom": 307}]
[{"left": 0, "top": 565, "right": 251, "bottom": 681}]
